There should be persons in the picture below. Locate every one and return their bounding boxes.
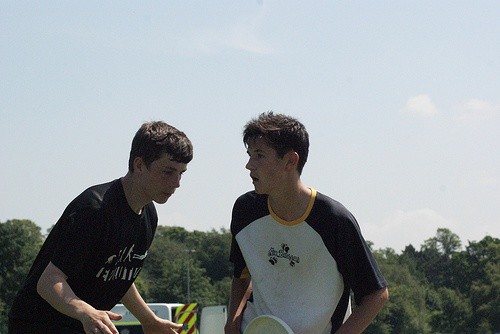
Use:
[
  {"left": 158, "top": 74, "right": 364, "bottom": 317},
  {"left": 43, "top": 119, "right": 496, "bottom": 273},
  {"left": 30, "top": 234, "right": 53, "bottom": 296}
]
[
  {"left": 223, "top": 109, "right": 389, "bottom": 333},
  {"left": 6, "top": 118, "right": 192, "bottom": 334}
]
